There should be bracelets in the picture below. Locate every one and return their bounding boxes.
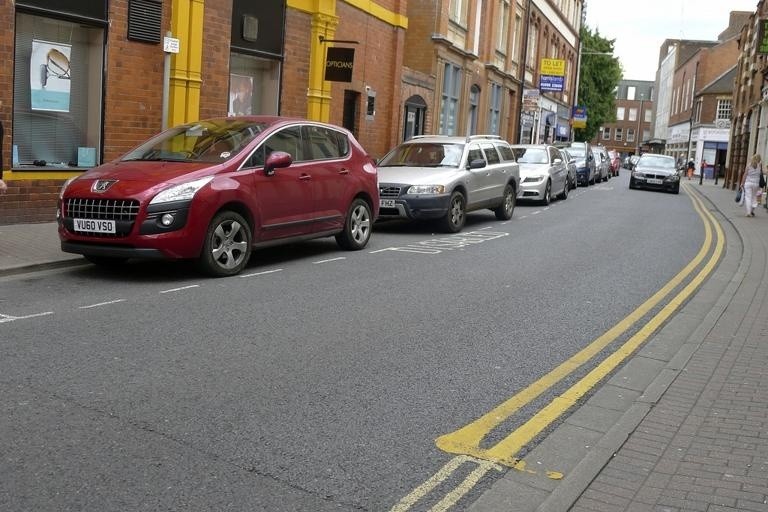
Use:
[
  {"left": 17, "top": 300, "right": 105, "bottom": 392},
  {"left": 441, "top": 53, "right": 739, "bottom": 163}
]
[{"left": 741, "top": 184, "right": 744, "bottom": 186}]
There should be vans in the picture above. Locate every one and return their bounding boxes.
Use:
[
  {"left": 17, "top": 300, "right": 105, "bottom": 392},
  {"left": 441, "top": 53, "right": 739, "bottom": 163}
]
[{"left": 376, "top": 135, "right": 519, "bottom": 233}]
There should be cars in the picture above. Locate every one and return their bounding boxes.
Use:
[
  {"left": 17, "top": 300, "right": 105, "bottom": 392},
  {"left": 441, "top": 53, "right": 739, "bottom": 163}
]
[
  {"left": 57, "top": 116, "right": 380, "bottom": 276},
  {"left": 622, "top": 153, "right": 680, "bottom": 194},
  {"left": 511, "top": 142, "right": 620, "bottom": 205}
]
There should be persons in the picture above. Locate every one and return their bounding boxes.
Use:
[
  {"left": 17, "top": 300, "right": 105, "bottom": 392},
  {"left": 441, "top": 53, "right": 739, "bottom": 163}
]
[
  {"left": 739, "top": 153, "right": 768, "bottom": 216},
  {"left": 701, "top": 159, "right": 708, "bottom": 179},
  {"left": 688, "top": 158, "right": 696, "bottom": 180}
]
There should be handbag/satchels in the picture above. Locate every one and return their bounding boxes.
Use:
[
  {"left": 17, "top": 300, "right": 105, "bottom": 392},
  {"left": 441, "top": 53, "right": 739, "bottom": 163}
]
[
  {"left": 736, "top": 188, "right": 744, "bottom": 206},
  {"left": 759, "top": 172, "right": 766, "bottom": 187}
]
